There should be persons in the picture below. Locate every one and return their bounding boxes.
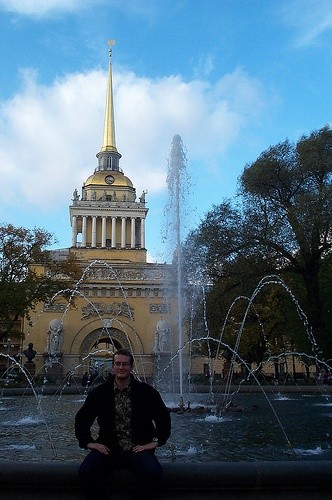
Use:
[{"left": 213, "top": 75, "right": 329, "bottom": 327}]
[
  {"left": 82, "top": 372, "right": 96, "bottom": 386},
  {"left": 315, "top": 352, "right": 328, "bottom": 386},
  {"left": 23, "top": 343, "right": 37, "bottom": 360},
  {"left": 75, "top": 349, "right": 171, "bottom": 499}
]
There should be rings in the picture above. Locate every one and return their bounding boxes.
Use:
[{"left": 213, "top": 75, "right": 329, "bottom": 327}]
[
  {"left": 105, "top": 449, "right": 107, "bottom": 451},
  {"left": 102, "top": 449, "right": 103, "bottom": 451}
]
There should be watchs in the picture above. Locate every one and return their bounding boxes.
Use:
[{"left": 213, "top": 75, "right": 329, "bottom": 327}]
[{"left": 152, "top": 437, "right": 158, "bottom": 442}]
